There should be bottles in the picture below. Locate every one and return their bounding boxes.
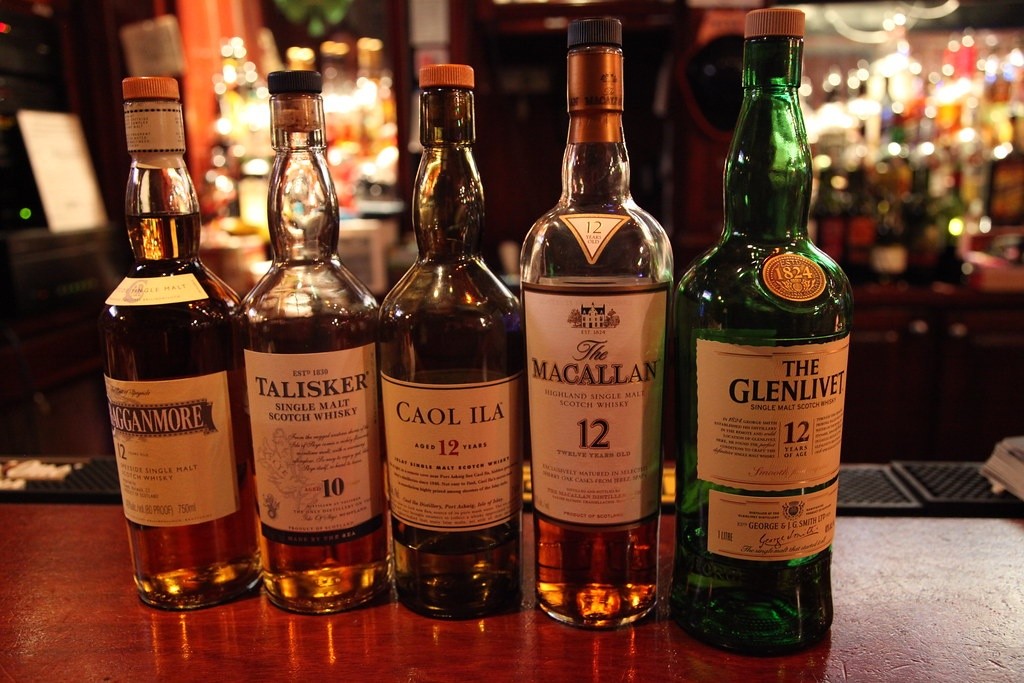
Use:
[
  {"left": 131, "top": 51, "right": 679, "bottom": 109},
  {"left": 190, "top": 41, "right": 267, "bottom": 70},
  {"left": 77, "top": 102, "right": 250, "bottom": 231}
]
[
  {"left": 378, "top": 62, "right": 522, "bottom": 621},
  {"left": 518, "top": 15, "right": 675, "bottom": 630},
  {"left": 219, "top": 36, "right": 397, "bottom": 222},
  {"left": 672, "top": 8, "right": 854, "bottom": 659},
  {"left": 233, "top": 71, "right": 396, "bottom": 615},
  {"left": 814, "top": 50, "right": 1024, "bottom": 280},
  {"left": 102, "top": 77, "right": 262, "bottom": 610}
]
